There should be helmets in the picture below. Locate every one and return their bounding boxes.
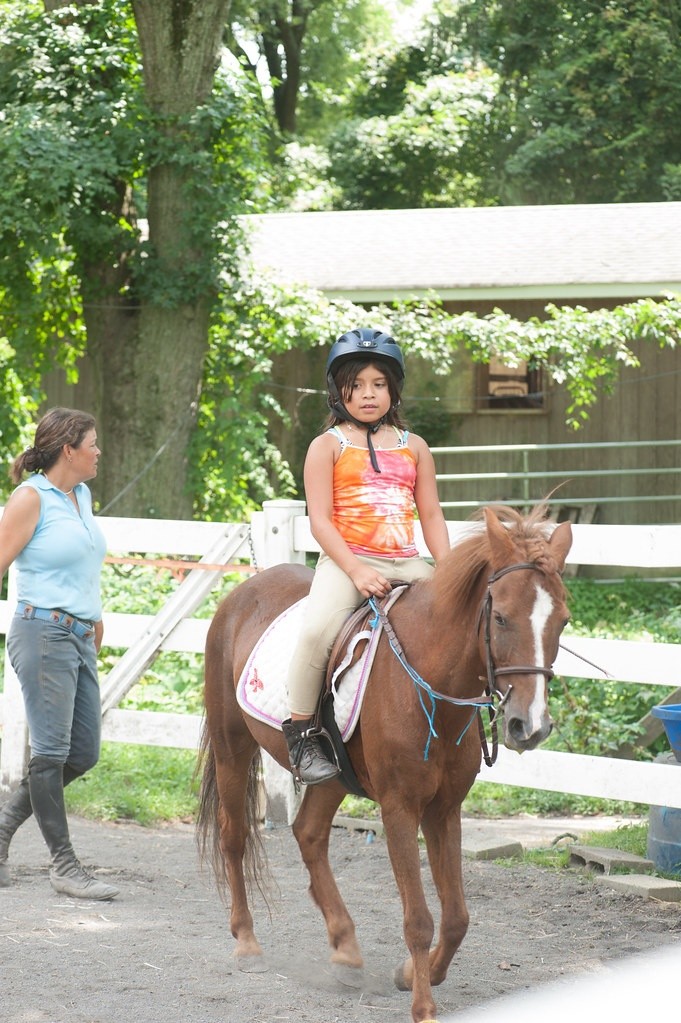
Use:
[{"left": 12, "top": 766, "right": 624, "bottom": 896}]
[{"left": 326, "top": 328, "right": 405, "bottom": 379}]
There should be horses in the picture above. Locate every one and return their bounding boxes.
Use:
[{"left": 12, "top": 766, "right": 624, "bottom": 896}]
[{"left": 192, "top": 507, "right": 571, "bottom": 1020}]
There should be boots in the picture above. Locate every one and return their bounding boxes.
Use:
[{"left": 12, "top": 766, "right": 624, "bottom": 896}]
[
  {"left": 28, "top": 756, "right": 120, "bottom": 900},
  {"left": 281, "top": 718, "right": 341, "bottom": 785},
  {"left": 0, "top": 761, "right": 85, "bottom": 888}
]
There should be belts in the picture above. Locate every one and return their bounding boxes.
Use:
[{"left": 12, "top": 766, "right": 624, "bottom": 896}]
[{"left": 15, "top": 602, "right": 94, "bottom": 640}]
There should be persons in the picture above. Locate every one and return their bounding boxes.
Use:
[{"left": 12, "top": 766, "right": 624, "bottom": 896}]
[
  {"left": 1, "top": 408, "right": 120, "bottom": 899},
  {"left": 280, "top": 327, "right": 451, "bottom": 785}
]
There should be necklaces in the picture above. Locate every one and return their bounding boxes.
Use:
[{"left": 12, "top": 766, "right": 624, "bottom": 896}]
[
  {"left": 65, "top": 489, "right": 72, "bottom": 494},
  {"left": 346, "top": 420, "right": 387, "bottom": 450}
]
